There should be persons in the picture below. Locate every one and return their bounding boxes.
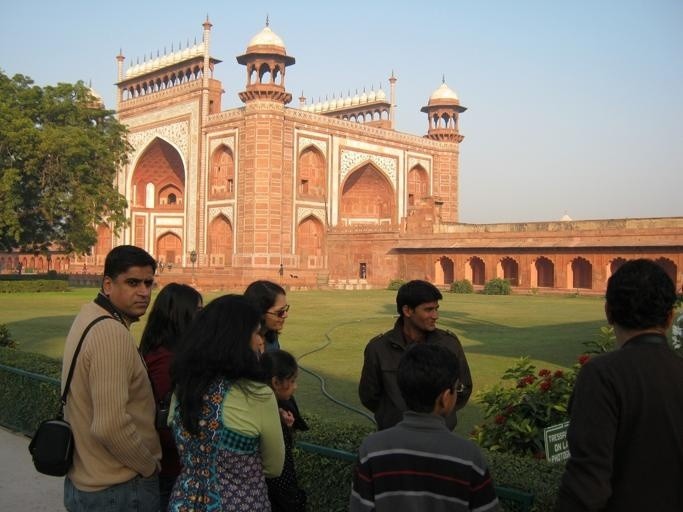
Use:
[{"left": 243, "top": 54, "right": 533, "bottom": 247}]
[
  {"left": 155, "top": 260, "right": 173, "bottom": 275},
  {"left": 257, "top": 350, "right": 310, "bottom": 512},
  {"left": 243, "top": 280, "right": 289, "bottom": 354},
  {"left": 289, "top": 274, "right": 298, "bottom": 278},
  {"left": 361, "top": 264, "right": 366, "bottom": 278},
  {"left": 138, "top": 283, "right": 203, "bottom": 512},
  {"left": 167, "top": 295, "right": 286, "bottom": 512},
  {"left": 61, "top": 246, "right": 163, "bottom": 512},
  {"left": 82, "top": 264, "right": 87, "bottom": 275},
  {"left": 348, "top": 343, "right": 504, "bottom": 512},
  {"left": 278, "top": 264, "right": 283, "bottom": 279},
  {"left": 557, "top": 259, "right": 683, "bottom": 512},
  {"left": 17, "top": 263, "right": 22, "bottom": 277},
  {"left": 359, "top": 280, "right": 473, "bottom": 432}
]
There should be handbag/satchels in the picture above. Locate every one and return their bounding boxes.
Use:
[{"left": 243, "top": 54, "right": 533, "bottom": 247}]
[{"left": 29, "top": 418, "right": 75, "bottom": 477}]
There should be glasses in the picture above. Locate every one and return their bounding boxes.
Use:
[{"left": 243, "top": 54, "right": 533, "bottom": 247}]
[
  {"left": 452, "top": 383, "right": 466, "bottom": 394},
  {"left": 265, "top": 305, "right": 290, "bottom": 318}
]
